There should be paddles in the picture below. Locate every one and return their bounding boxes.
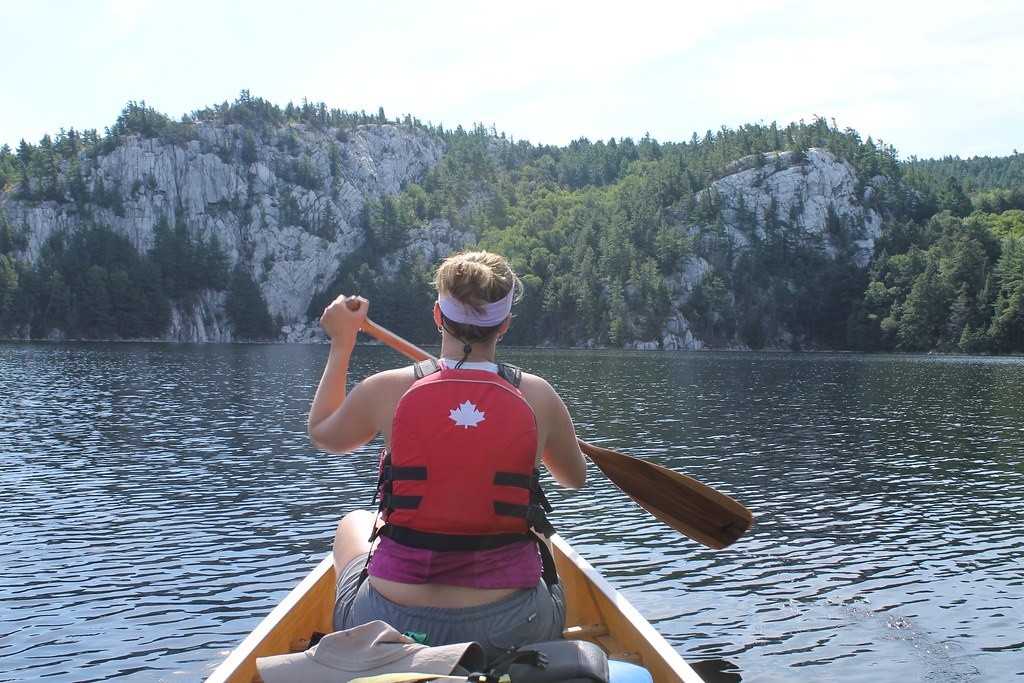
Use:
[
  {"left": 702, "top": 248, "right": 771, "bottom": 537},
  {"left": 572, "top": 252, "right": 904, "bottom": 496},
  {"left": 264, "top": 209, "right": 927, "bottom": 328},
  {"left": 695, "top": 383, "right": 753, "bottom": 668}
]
[{"left": 343, "top": 294, "right": 754, "bottom": 550}]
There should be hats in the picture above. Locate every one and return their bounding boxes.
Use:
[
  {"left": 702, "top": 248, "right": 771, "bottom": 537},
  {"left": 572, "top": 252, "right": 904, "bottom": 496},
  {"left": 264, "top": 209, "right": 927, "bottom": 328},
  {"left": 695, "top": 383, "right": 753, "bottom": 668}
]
[{"left": 254, "top": 618, "right": 490, "bottom": 682}]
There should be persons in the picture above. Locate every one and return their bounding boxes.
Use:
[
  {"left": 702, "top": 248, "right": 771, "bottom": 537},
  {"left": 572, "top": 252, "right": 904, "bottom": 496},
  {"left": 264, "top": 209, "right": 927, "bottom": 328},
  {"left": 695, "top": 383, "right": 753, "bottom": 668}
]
[{"left": 306, "top": 249, "right": 587, "bottom": 669}]
